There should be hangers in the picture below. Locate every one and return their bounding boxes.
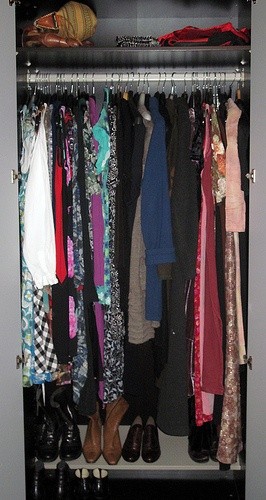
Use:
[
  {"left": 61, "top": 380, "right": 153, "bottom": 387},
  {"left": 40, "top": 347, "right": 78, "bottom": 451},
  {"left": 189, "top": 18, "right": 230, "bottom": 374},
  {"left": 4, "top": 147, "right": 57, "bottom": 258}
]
[{"left": 22, "top": 69, "right": 244, "bottom": 116}]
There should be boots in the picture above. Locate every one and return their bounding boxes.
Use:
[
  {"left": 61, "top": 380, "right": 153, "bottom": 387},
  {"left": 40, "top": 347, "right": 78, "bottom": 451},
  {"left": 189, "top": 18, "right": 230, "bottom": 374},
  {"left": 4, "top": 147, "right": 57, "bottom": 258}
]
[
  {"left": 31, "top": 462, "right": 45, "bottom": 500},
  {"left": 91, "top": 468, "right": 108, "bottom": 500},
  {"left": 188, "top": 395, "right": 209, "bottom": 462},
  {"left": 82, "top": 402, "right": 102, "bottom": 463},
  {"left": 210, "top": 394, "right": 223, "bottom": 462},
  {"left": 37, "top": 406, "right": 57, "bottom": 461},
  {"left": 72, "top": 469, "right": 90, "bottom": 500},
  {"left": 56, "top": 462, "right": 69, "bottom": 500},
  {"left": 102, "top": 396, "right": 129, "bottom": 465},
  {"left": 58, "top": 406, "right": 82, "bottom": 461}
]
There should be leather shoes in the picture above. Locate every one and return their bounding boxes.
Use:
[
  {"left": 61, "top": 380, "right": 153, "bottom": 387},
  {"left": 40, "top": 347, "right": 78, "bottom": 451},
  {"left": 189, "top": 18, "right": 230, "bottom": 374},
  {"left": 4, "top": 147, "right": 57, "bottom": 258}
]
[
  {"left": 122, "top": 414, "right": 143, "bottom": 462},
  {"left": 142, "top": 416, "right": 161, "bottom": 463}
]
